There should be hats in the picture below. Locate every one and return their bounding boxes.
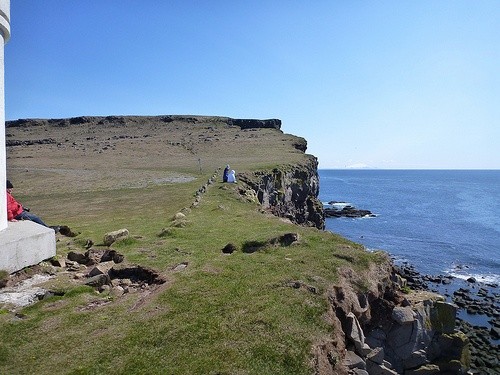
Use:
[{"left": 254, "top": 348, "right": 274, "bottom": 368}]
[{"left": 7, "top": 180, "right": 14, "bottom": 188}]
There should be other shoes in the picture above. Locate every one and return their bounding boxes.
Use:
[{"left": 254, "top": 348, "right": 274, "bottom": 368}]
[{"left": 49, "top": 225, "right": 60, "bottom": 234}]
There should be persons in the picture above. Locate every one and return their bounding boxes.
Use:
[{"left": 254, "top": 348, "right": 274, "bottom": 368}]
[
  {"left": 231, "top": 168, "right": 237, "bottom": 183},
  {"left": 6, "top": 179, "right": 61, "bottom": 234},
  {"left": 223, "top": 164, "right": 230, "bottom": 182}
]
